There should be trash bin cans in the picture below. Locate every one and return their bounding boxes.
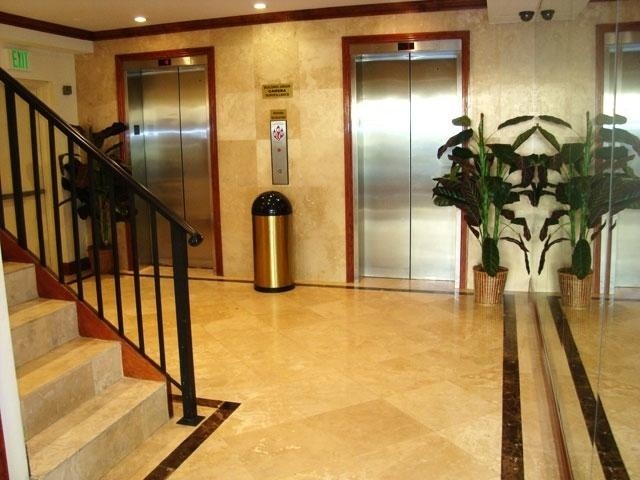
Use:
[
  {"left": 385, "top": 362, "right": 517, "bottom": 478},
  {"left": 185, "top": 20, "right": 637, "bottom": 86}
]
[{"left": 252, "top": 191, "right": 294, "bottom": 293}]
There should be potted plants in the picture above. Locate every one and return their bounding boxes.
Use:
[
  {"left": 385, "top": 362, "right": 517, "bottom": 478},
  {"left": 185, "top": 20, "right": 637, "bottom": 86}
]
[
  {"left": 431, "top": 112, "right": 538, "bottom": 306},
  {"left": 538, "top": 110, "right": 639, "bottom": 310},
  {"left": 59, "top": 121, "right": 130, "bottom": 276}
]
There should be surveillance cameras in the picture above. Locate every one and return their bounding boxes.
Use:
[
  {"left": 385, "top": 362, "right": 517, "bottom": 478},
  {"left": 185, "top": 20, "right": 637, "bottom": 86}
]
[
  {"left": 521, "top": 12, "right": 534, "bottom": 23},
  {"left": 542, "top": 10, "right": 555, "bottom": 21}
]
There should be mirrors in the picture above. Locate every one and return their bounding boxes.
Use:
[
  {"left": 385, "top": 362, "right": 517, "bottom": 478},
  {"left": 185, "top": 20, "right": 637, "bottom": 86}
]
[{"left": 535, "top": 0, "right": 639, "bottom": 479}]
